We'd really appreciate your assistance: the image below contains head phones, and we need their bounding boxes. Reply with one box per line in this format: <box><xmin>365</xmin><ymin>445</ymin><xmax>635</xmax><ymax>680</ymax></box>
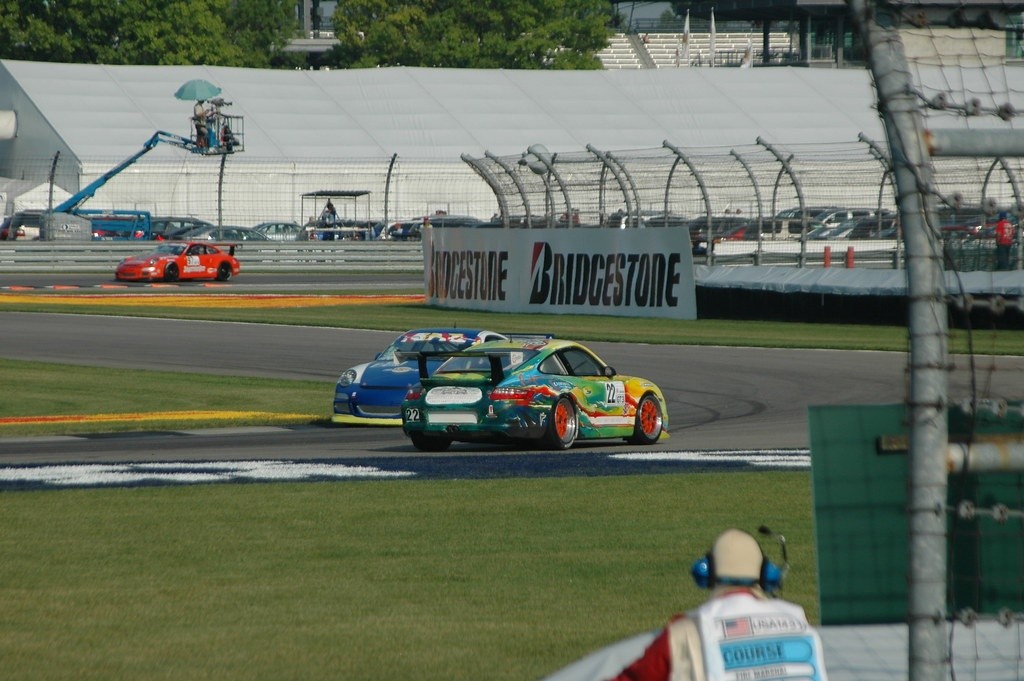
<box><xmin>690</xmin><ymin>529</ymin><xmax>781</xmax><ymax>592</ymax></box>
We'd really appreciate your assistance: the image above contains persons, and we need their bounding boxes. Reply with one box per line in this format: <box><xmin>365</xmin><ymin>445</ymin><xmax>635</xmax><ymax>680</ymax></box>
<box><xmin>421</xmin><ymin>217</ymin><xmax>433</xmax><ymax>243</ymax></box>
<box><xmin>326</xmin><ymin>198</ymin><xmax>336</xmax><ymax>228</ymax></box>
<box><xmin>611</xmin><ymin>527</ymin><xmax>827</xmax><ymax>681</ymax></box>
<box><xmin>996</xmin><ymin>212</ymin><xmax>1014</xmax><ymax>270</ymax></box>
<box><xmin>194</xmin><ymin>99</ymin><xmax>206</xmax><ymax>148</ymax></box>
<box><xmin>644</xmin><ymin>33</ymin><xmax>651</xmax><ymax>43</ymax></box>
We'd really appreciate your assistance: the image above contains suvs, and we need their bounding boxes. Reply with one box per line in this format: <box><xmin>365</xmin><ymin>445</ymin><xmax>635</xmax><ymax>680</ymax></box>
<box><xmin>0</xmin><ymin>209</ymin><xmax>45</xmax><ymax>241</ymax></box>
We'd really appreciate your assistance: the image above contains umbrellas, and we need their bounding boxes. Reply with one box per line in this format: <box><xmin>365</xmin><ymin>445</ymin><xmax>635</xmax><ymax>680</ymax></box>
<box><xmin>174</xmin><ymin>80</ymin><xmax>221</xmax><ymax>101</ymax></box>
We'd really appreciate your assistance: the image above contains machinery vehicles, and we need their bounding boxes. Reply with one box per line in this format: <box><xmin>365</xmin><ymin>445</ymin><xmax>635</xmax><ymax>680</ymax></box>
<box><xmin>53</xmin><ymin>97</ymin><xmax>244</xmax><ymax>243</ymax></box>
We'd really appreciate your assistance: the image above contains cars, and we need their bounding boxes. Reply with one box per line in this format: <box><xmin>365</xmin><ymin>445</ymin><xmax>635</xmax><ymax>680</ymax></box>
<box><xmin>380</xmin><ymin>213</ymin><xmax>518</xmax><ymax>243</ymax></box>
<box><xmin>150</xmin><ymin>213</ymin><xmax>302</xmax><ymax>243</ymax></box>
<box><xmin>607</xmin><ymin>204</ymin><xmax>1019</xmax><ymax>253</ymax></box>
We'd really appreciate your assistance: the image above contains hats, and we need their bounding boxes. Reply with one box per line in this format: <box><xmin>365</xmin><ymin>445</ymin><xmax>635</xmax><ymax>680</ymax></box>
<box><xmin>1000</xmin><ymin>212</ymin><xmax>1007</xmax><ymax>219</ymax></box>
<box><xmin>709</xmin><ymin>527</ymin><xmax>763</xmax><ymax>585</ymax></box>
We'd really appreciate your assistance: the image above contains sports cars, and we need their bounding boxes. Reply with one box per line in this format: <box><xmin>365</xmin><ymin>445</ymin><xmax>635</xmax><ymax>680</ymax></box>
<box><xmin>115</xmin><ymin>242</ymin><xmax>243</xmax><ymax>283</ymax></box>
<box><xmin>331</xmin><ymin>325</ymin><xmax>526</xmax><ymax>427</ymax></box>
<box><xmin>392</xmin><ymin>336</ymin><xmax>669</xmax><ymax>453</ymax></box>
